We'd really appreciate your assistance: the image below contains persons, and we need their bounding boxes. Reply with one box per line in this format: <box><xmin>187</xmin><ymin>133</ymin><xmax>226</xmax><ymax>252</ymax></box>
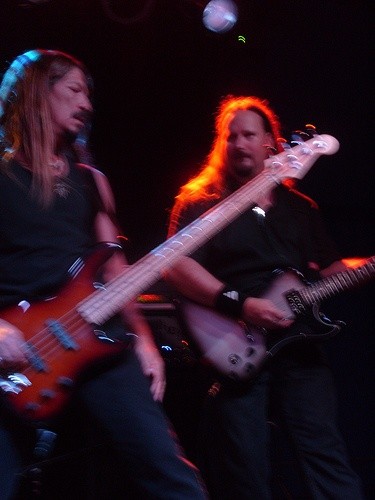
<box><xmin>162</xmin><ymin>94</ymin><xmax>375</xmax><ymax>500</ymax></box>
<box><xmin>1</xmin><ymin>52</ymin><xmax>206</xmax><ymax>500</ymax></box>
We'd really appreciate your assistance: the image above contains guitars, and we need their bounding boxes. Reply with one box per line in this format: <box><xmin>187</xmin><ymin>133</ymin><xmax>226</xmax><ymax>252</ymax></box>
<box><xmin>176</xmin><ymin>252</ymin><xmax>375</xmax><ymax>386</ymax></box>
<box><xmin>1</xmin><ymin>125</ymin><xmax>340</xmax><ymax>416</ymax></box>
